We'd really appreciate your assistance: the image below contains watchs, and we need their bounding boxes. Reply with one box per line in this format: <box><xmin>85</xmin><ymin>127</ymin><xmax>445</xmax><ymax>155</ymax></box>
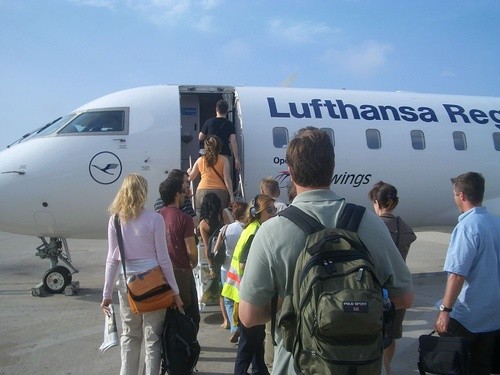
<box><xmin>439</xmin><ymin>304</ymin><xmax>453</xmax><ymax>312</ymax></box>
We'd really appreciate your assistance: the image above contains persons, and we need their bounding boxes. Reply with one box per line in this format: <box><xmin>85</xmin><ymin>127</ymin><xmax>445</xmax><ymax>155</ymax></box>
<box><xmin>100</xmin><ymin>174</ymin><xmax>184</xmax><ymax>375</ymax></box>
<box><xmin>154</xmin><ymin>98</ymin><xmax>297</xmax><ymax>374</ymax></box>
<box><xmin>436</xmin><ymin>172</ymin><xmax>500</xmax><ymax>375</ymax></box>
<box><xmin>237</xmin><ymin>124</ymin><xmax>414</xmax><ymax>375</ymax></box>
<box><xmin>369</xmin><ymin>180</ymin><xmax>417</xmax><ymax>374</ymax></box>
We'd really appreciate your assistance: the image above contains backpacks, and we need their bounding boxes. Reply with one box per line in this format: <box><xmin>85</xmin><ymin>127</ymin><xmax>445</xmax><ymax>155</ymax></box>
<box><xmin>161</xmin><ymin>304</ymin><xmax>200</xmax><ymax>375</ymax></box>
<box><xmin>274</xmin><ymin>203</ymin><xmax>396</xmax><ymax>375</ymax></box>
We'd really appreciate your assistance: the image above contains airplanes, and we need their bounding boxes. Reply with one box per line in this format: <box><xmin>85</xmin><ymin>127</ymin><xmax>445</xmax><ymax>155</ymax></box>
<box><xmin>0</xmin><ymin>84</ymin><xmax>500</xmax><ymax>298</ymax></box>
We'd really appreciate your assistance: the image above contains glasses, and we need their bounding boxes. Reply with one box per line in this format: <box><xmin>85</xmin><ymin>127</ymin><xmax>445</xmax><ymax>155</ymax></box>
<box><xmin>266</xmin><ymin>207</ymin><xmax>277</xmax><ymax>214</ymax></box>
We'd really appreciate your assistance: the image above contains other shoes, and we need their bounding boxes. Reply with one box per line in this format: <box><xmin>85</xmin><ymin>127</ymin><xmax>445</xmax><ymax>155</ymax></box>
<box><xmin>230</xmin><ymin>329</ymin><xmax>241</xmax><ymax>343</ymax></box>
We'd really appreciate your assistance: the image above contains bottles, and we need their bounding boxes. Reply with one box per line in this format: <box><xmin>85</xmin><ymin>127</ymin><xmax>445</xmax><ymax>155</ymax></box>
<box><xmin>211</xmin><ymin>237</ymin><xmax>217</xmax><ymax>253</ymax></box>
<box><xmin>382</xmin><ymin>288</ymin><xmax>393</xmax><ymax>349</ymax></box>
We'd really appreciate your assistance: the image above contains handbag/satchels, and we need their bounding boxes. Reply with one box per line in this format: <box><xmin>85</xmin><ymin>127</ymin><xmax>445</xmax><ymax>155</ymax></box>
<box><xmin>125</xmin><ymin>265</ymin><xmax>176</xmax><ymax>316</ymax></box>
<box><xmin>213</xmin><ymin>223</ymin><xmax>229</xmax><ymax>266</ymax></box>
<box><xmin>222</xmin><ymin>207</ymin><xmax>235</xmax><ymax>224</ymax></box>
<box><xmin>418</xmin><ymin>328</ymin><xmax>470</xmax><ymax>375</ymax></box>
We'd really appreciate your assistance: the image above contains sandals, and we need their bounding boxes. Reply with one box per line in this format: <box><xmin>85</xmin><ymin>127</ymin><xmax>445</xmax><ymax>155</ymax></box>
<box><xmin>223</xmin><ymin>320</ymin><xmax>230</xmax><ymax>328</ymax></box>
